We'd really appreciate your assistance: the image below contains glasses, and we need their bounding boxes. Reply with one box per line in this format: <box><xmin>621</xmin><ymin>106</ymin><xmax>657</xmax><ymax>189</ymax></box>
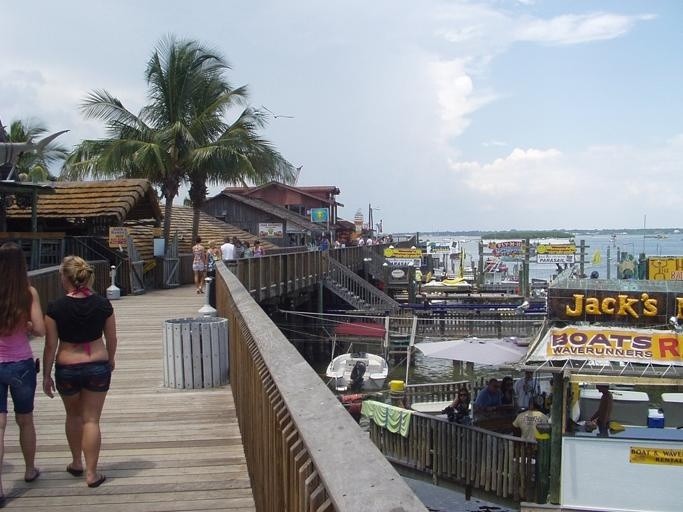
<box><xmin>459</xmin><ymin>393</ymin><xmax>466</xmax><ymax>396</ymax></box>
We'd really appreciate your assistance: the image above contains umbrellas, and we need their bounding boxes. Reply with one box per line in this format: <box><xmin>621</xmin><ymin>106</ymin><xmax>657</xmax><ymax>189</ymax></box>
<box><xmin>414</xmin><ymin>338</ymin><xmax>522</xmax><ymax>426</ymax></box>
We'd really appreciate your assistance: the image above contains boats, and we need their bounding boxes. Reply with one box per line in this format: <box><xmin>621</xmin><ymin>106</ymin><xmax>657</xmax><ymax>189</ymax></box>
<box><xmin>393</xmin><ymin>257</ymin><xmax>549</xmax><ymax>303</ymax></box>
<box><xmin>326</xmin><ymin>352</ymin><xmax>388</xmax><ymax>392</ymax></box>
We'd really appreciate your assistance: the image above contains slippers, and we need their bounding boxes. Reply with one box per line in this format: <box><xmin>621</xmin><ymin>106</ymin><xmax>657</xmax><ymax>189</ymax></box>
<box><xmin>66</xmin><ymin>463</ymin><xmax>83</xmax><ymax>475</ymax></box>
<box><xmin>88</xmin><ymin>474</ymin><xmax>106</xmax><ymax>488</ymax></box>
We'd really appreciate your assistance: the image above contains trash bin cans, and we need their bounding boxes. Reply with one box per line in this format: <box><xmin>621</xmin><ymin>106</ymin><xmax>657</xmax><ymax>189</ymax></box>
<box><xmin>164</xmin><ymin>317</ymin><xmax>230</xmax><ymax>389</ymax></box>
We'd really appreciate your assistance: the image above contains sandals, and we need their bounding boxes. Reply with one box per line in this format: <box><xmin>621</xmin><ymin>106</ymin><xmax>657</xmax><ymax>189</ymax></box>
<box><xmin>25</xmin><ymin>467</ymin><xmax>39</xmax><ymax>483</ymax></box>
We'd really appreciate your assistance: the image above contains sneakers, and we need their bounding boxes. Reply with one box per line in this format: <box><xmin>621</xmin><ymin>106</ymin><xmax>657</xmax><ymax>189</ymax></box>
<box><xmin>196</xmin><ymin>286</ymin><xmax>203</xmax><ymax>293</ymax></box>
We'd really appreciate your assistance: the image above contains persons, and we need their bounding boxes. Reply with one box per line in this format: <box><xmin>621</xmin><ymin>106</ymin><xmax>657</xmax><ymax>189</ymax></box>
<box><xmin>192</xmin><ymin>236</ymin><xmax>264</xmax><ymax>294</ymax></box>
<box><xmin>441</xmin><ymin>371</ymin><xmax>613</xmax><ymax>440</ymax></box>
<box><xmin>318</xmin><ymin>234</ymin><xmax>393</xmax><ymax>250</ymax></box>
<box><xmin>0</xmin><ymin>242</ymin><xmax>117</xmax><ymax>502</ymax></box>
